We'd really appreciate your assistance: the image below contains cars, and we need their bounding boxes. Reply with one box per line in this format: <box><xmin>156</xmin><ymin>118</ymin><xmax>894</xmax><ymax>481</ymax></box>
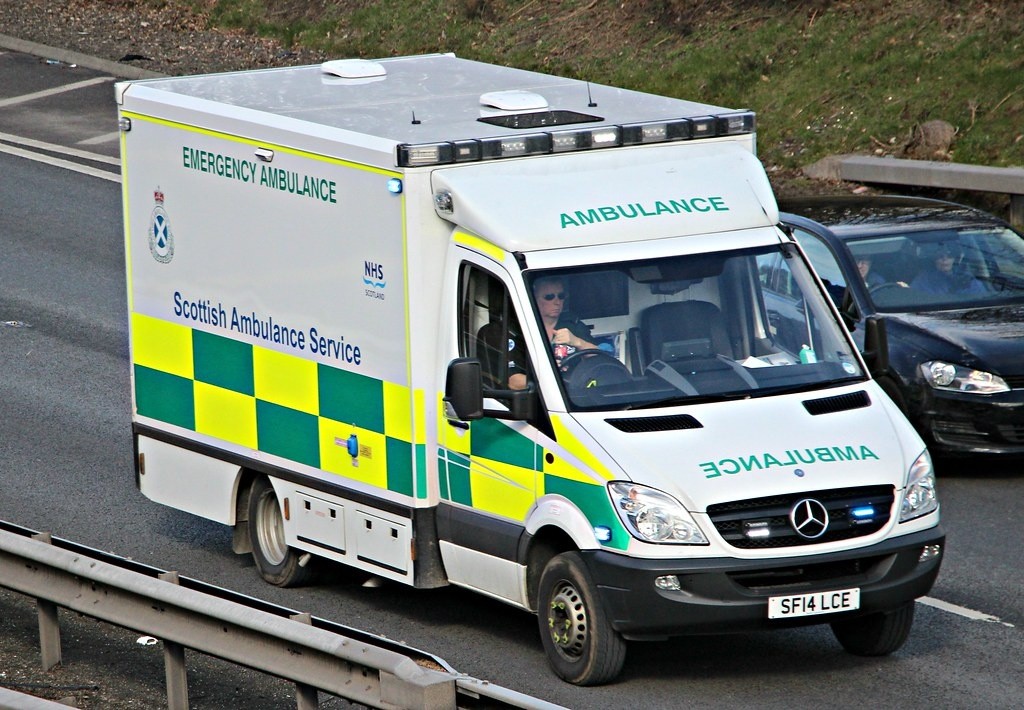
<box><xmin>756</xmin><ymin>195</ymin><xmax>1024</xmax><ymax>479</ymax></box>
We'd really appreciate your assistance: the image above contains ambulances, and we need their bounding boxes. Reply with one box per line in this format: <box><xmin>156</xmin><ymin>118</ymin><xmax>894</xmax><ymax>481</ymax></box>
<box><xmin>115</xmin><ymin>53</ymin><xmax>946</xmax><ymax>686</ymax></box>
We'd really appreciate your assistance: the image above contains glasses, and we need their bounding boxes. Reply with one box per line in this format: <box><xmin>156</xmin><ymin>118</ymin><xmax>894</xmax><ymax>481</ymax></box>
<box><xmin>543</xmin><ymin>292</ymin><xmax>567</xmax><ymax>301</ymax></box>
<box><xmin>934</xmin><ymin>252</ymin><xmax>955</xmax><ymax>260</ymax></box>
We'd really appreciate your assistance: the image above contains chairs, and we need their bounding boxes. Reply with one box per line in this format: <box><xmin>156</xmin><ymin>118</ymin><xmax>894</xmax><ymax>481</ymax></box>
<box><xmin>477</xmin><ymin>319</ymin><xmax>592</xmax><ymax>389</ymax></box>
<box><xmin>630</xmin><ymin>300</ymin><xmax>733</xmax><ymax>378</ymax></box>
<box><xmin>892</xmin><ymin>246</ymin><xmax>929</xmax><ymax>284</ymax></box>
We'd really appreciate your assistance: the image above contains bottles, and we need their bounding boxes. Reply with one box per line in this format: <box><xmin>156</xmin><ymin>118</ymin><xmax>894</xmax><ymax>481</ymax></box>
<box><xmin>555</xmin><ymin>344</ymin><xmax>567</xmax><ymax>363</ymax></box>
<box><xmin>799</xmin><ymin>344</ymin><xmax>817</xmax><ymax>364</ymax></box>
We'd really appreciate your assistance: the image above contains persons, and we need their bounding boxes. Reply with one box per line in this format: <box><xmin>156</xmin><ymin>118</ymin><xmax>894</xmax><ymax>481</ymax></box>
<box><xmin>826</xmin><ymin>244</ymin><xmax>987</xmax><ymax>305</ymax></box>
<box><xmin>507</xmin><ymin>277</ymin><xmax>616</xmax><ymax>391</ymax></box>
